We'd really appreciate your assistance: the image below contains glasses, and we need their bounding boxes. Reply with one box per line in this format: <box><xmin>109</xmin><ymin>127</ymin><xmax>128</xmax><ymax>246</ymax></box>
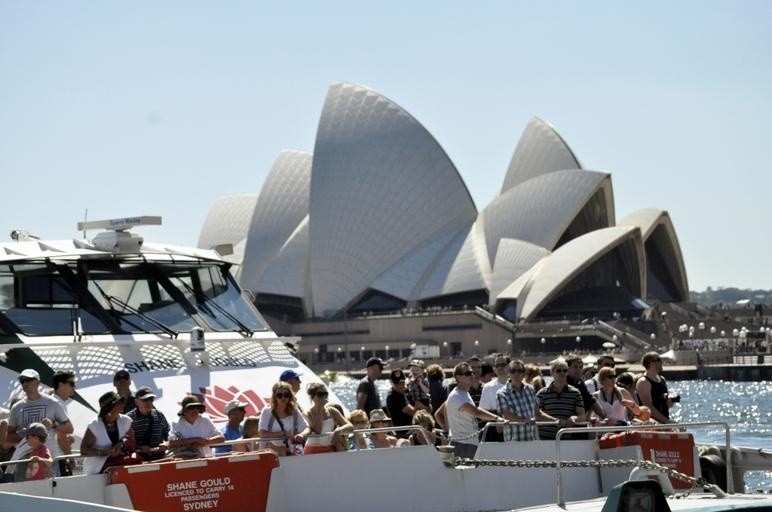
<box><xmin>276</xmin><ymin>392</ymin><xmax>291</xmax><ymax>398</ymax></box>
<box><xmin>462</xmin><ymin>363</ymin><xmax>569</xmax><ymax>378</ymax></box>
<box><xmin>317</xmin><ymin>391</ymin><xmax>329</xmax><ymax>397</ymax></box>
<box><xmin>605</xmin><ymin>362</ymin><xmax>614</xmax><ymax>368</ymax></box>
<box><xmin>607</xmin><ymin>374</ymin><xmax>617</xmax><ymax>379</ymax></box>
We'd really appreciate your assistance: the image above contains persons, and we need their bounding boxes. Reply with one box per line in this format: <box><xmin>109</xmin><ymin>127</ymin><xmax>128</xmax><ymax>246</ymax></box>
<box><xmin>353</xmin><ymin>351</ymin><xmax>687</xmax><ymax>461</ymax></box>
<box><xmin>0</xmin><ymin>370</ymin><xmax>354</xmax><ymax>484</ymax></box>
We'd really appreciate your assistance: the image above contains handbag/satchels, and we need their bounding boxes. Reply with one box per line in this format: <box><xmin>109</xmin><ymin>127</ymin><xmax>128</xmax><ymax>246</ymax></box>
<box><xmin>107</xmin><ymin>436</ymin><xmax>141</xmax><ymax>465</ymax></box>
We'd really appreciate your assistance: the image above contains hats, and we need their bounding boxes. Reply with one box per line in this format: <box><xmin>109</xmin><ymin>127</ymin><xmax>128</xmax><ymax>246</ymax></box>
<box><xmin>98</xmin><ymin>370</ymin><xmax>157</xmax><ymax>417</ymax></box>
<box><xmin>370</xmin><ymin>409</ymin><xmax>391</xmax><ymax>423</ymax></box>
<box><xmin>225</xmin><ymin>400</ymin><xmax>248</xmax><ymax>414</ymax></box>
<box><xmin>177</xmin><ymin>395</ymin><xmax>205</xmax><ymax>416</ymax></box>
<box><xmin>17</xmin><ymin>367</ymin><xmax>41</xmax><ymax>384</ymax></box>
<box><xmin>280</xmin><ymin>370</ymin><xmax>302</xmax><ymax>382</ymax></box>
<box><xmin>19</xmin><ymin>423</ymin><xmax>48</xmax><ymax>438</ymax></box>
<box><xmin>367</xmin><ymin>357</ymin><xmax>424</xmax><ymax>384</ymax></box>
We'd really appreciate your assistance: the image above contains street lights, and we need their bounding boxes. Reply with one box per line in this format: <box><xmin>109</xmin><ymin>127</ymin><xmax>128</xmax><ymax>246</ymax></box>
<box><xmin>333</xmin><ymin>344</ymin><xmax>342</xmax><ymax>363</ymax></box>
<box><xmin>648</xmin><ymin>332</ymin><xmax>656</xmax><ymax>351</ymax></box>
<box><xmin>659</xmin><ymin>310</ymin><xmax>668</xmax><ymax>324</ymax></box>
<box><xmin>384</xmin><ymin>344</ymin><xmax>390</xmax><ymax>360</ymax></box>
<box><xmin>408</xmin><ymin>341</ymin><xmax>416</xmax><ymax>359</ymax></box>
<box><xmin>358</xmin><ymin>344</ymin><xmax>366</xmax><ymax>361</ymax></box>
<box><xmin>673</xmin><ymin>317</ymin><xmax>771</xmax><ymax>352</ymax></box>
<box><xmin>541</xmin><ymin>335</ymin><xmax>546</xmax><ymax>356</ymax></box>
<box><xmin>444</xmin><ymin>341</ymin><xmax>448</xmax><ymax>358</ymax></box>
<box><xmin>473</xmin><ymin>339</ymin><xmax>480</xmax><ymax>357</ymax></box>
<box><xmin>612</xmin><ymin>332</ymin><xmax>618</xmax><ymax>351</ymax></box>
<box><xmin>507</xmin><ymin>337</ymin><xmax>512</xmax><ymax>355</ymax></box>
<box><xmin>313</xmin><ymin>346</ymin><xmax>319</xmax><ymax>362</ymax></box>
<box><xmin>574</xmin><ymin>332</ymin><xmax>581</xmax><ymax>354</ymax></box>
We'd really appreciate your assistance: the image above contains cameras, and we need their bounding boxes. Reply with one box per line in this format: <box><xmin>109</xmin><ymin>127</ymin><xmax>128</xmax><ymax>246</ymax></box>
<box><xmin>663</xmin><ymin>392</ymin><xmax>681</xmax><ymax>402</ymax></box>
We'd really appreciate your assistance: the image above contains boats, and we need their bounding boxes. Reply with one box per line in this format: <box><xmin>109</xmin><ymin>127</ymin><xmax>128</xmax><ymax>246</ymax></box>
<box><xmin>0</xmin><ymin>212</ymin><xmax>772</xmax><ymax>512</ymax></box>
